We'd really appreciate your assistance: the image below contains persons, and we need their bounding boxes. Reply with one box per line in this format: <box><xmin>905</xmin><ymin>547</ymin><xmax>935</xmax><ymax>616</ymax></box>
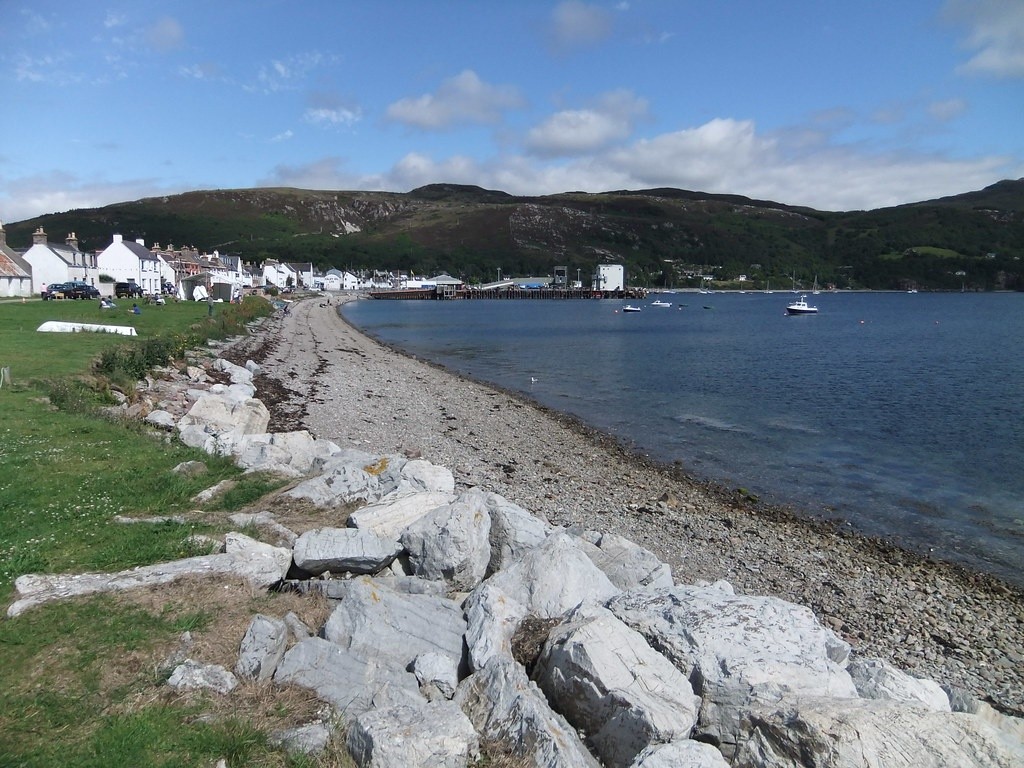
<box><xmin>207</xmin><ymin>294</ymin><xmax>213</xmax><ymax>317</ymax></box>
<box><xmin>100</xmin><ymin>295</ymin><xmax>116</xmax><ymax>309</ymax></box>
<box><xmin>232</xmin><ymin>288</ymin><xmax>244</xmax><ymax>306</ymax></box>
<box><xmin>128</xmin><ymin>302</ymin><xmax>141</xmax><ymax>314</ymax></box>
<box><xmin>460</xmin><ymin>284</ymin><xmax>648</xmax><ymax>299</ymax></box>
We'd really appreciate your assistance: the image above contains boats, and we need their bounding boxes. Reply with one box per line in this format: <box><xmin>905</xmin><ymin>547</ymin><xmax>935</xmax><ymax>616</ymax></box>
<box><xmin>785</xmin><ymin>295</ymin><xmax>818</xmax><ymax>316</ymax></box>
<box><xmin>651</xmin><ymin>300</ymin><xmax>673</xmax><ymax>308</ymax></box>
<box><xmin>37</xmin><ymin>321</ymin><xmax>139</xmax><ymax>337</ymax></box>
<box><xmin>621</xmin><ymin>305</ymin><xmax>642</xmax><ymax>312</ymax></box>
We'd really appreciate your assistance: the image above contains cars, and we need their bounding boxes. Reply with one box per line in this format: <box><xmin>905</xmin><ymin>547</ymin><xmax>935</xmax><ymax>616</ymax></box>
<box><xmin>46</xmin><ymin>283</ymin><xmax>72</xmax><ymax>298</ymax></box>
<box><xmin>69</xmin><ymin>285</ymin><xmax>100</xmax><ymax>298</ymax></box>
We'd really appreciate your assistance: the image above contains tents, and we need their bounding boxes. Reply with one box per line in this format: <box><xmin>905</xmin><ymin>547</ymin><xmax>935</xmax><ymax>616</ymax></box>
<box><xmin>175</xmin><ymin>271</ymin><xmax>240</xmax><ymax>303</ymax></box>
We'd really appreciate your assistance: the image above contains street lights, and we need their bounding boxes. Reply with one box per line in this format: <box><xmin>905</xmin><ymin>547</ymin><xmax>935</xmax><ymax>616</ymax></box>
<box><xmin>497</xmin><ymin>268</ymin><xmax>501</xmax><ymax>281</ymax></box>
<box><xmin>576</xmin><ymin>268</ymin><xmax>581</xmax><ymax>291</ymax></box>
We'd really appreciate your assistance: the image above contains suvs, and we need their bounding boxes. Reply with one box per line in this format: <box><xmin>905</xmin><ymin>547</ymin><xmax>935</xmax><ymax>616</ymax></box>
<box><xmin>116</xmin><ymin>282</ymin><xmax>143</xmax><ymax>299</ymax></box>
<box><xmin>64</xmin><ymin>281</ymin><xmax>86</xmax><ymax>291</ymax></box>
